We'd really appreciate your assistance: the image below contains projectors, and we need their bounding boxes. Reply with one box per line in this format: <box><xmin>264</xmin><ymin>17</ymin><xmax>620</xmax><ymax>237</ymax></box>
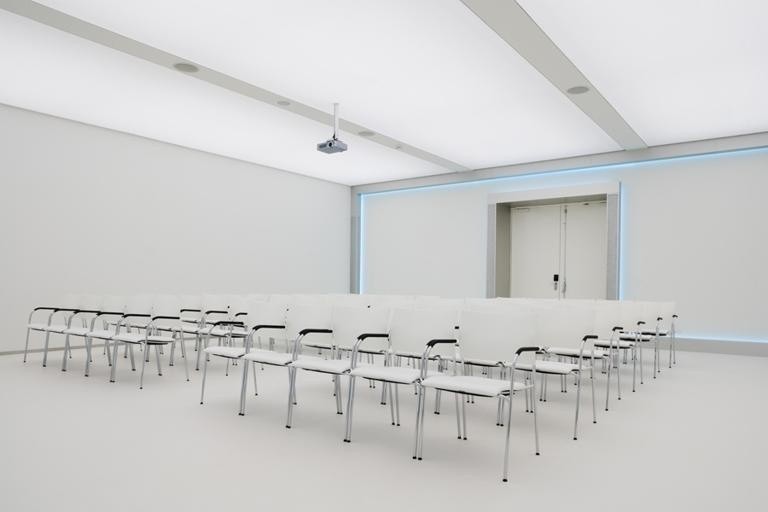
<box><xmin>316</xmin><ymin>140</ymin><xmax>347</xmax><ymax>154</ymax></box>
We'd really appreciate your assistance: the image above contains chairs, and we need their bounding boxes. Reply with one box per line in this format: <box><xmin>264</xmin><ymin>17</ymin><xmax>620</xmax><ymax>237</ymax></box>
<box><xmin>21</xmin><ymin>294</ymin><xmax>679</xmax><ymax>483</ymax></box>
